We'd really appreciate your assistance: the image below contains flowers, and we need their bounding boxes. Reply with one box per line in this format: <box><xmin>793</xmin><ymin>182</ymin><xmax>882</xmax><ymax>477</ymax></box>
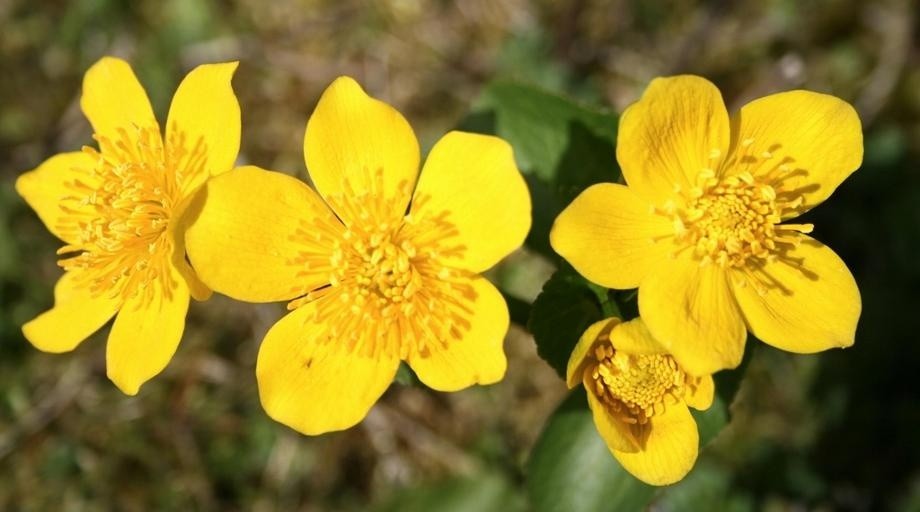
<box><xmin>10</xmin><ymin>53</ymin><xmax>906</xmax><ymax>511</ymax></box>
